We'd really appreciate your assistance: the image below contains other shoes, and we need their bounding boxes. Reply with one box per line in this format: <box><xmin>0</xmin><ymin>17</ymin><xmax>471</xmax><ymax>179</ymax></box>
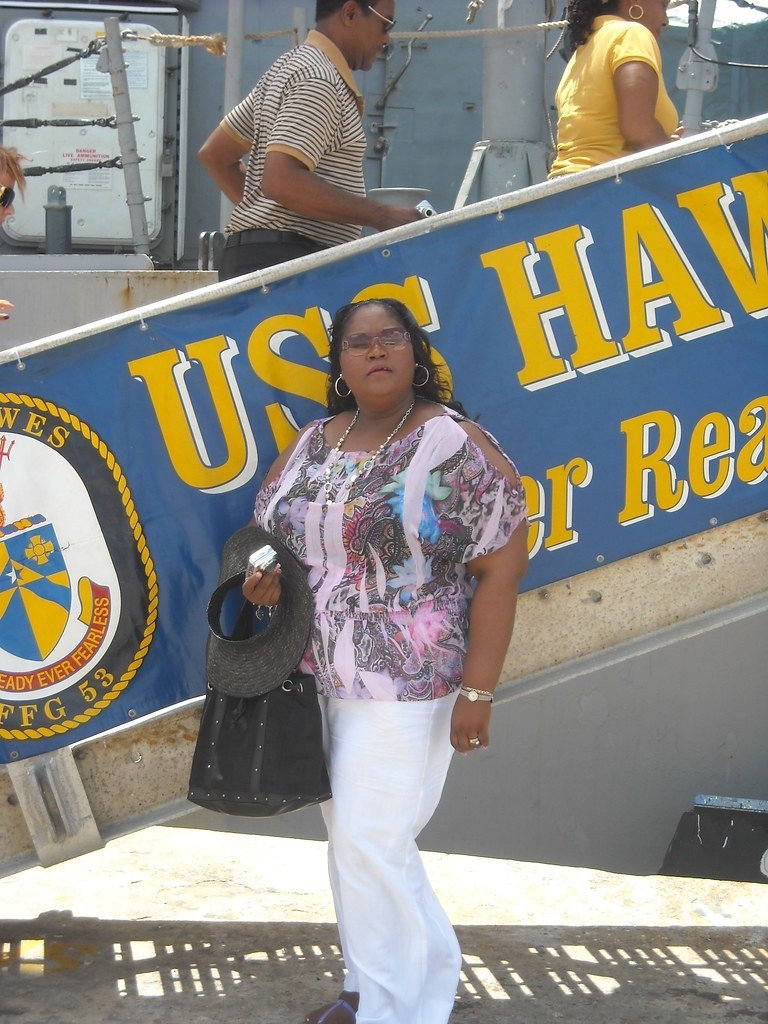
<box><xmin>302</xmin><ymin>990</ymin><xmax>360</xmax><ymax>1024</ymax></box>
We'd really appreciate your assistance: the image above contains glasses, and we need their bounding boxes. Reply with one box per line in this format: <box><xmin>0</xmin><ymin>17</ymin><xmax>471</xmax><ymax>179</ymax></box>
<box><xmin>0</xmin><ymin>183</ymin><xmax>15</xmax><ymax>209</ymax></box>
<box><xmin>357</xmin><ymin>0</ymin><xmax>397</xmax><ymax>32</ymax></box>
<box><xmin>341</xmin><ymin>327</ymin><xmax>411</xmax><ymax>356</ymax></box>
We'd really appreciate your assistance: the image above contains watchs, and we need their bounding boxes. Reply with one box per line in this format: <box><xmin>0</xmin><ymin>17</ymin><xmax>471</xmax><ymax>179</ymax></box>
<box><xmin>459</xmin><ymin>691</ymin><xmax>495</xmax><ymax>702</ymax></box>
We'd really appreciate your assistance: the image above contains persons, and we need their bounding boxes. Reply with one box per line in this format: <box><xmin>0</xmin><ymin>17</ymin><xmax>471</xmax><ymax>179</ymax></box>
<box><xmin>242</xmin><ymin>298</ymin><xmax>532</xmax><ymax>1024</ymax></box>
<box><xmin>0</xmin><ymin>146</ymin><xmax>26</xmax><ymax>321</ymax></box>
<box><xmin>199</xmin><ymin>0</ymin><xmax>422</xmax><ymax>282</ymax></box>
<box><xmin>547</xmin><ymin>0</ymin><xmax>684</xmax><ymax>180</ymax></box>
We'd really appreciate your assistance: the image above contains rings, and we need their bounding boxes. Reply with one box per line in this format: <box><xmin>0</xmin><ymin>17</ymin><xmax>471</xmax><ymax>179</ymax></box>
<box><xmin>469</xmin><ymin>736</ymin><xmax>480</xmax><ymax>745</ymax></box>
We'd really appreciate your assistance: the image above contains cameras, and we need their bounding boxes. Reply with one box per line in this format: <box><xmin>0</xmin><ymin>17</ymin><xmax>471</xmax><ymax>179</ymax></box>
<box><xmin>245</xmin><ymin>545</ymin><xmax>277</xmax><ymax>581</ymax></box>
<box><xmin>416</xmin><ymin>200</ymin><xmax>437</xmax><ymax>218</ymax></box>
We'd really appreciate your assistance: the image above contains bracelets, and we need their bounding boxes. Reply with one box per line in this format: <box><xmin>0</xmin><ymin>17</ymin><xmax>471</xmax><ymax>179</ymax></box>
<box><xmin>461</xmin><ymin>686</ymin><xmax>491</xmax><ymax>696</ymax></box>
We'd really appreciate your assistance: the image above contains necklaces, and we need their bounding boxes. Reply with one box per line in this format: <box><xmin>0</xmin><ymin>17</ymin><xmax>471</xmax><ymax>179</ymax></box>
<box><xmin>324</xmin><ymin>400</ymin><xmax>416</xmax><ymax>510</ymax></box>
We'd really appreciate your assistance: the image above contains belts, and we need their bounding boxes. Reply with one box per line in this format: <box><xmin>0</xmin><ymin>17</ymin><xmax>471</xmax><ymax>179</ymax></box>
<box><xmin>221</xmin><ymin>229</ymin><xmax>328</xmax><ymax>252</ymax></box>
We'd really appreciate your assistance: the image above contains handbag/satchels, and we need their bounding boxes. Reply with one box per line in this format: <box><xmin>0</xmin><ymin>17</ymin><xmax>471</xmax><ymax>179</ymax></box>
<box><xmin>187</xmin><ymin>598</ymin><xmax>332</xmax><ymax>817</ymax></box>
